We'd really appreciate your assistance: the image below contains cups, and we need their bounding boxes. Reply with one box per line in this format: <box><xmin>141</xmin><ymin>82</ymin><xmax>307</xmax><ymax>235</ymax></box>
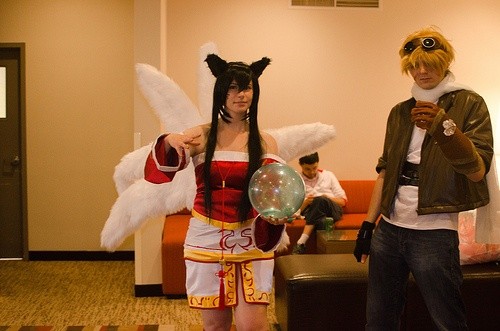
<box><xmin>416</xmin><ymin>100</ymin><xmax>435</xmax><ymax>129</ymax></box>
<box><xmin>324</xmin><ymin>218</ymin><xmax>333</xmax><ymax>233</ymax></box>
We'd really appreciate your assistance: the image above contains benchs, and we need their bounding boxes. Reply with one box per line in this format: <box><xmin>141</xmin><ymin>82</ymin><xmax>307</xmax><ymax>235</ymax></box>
<box><xmin>274</xmin><ymin>255</ymin><xmax>500</xmax><ymax>331</ymax></box>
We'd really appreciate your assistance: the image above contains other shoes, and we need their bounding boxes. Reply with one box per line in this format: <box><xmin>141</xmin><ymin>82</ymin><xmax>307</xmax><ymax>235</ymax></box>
<box><xmin>291</xmin><ymin>243</ymin><xmax>305</xmax><ymax>254</ymax></box>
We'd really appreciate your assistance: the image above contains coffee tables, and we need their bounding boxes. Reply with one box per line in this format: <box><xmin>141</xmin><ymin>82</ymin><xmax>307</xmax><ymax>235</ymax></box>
<box><xmin>316</xmin><ymin>230</ymin><xmax>362</xmax><ymax>255</ymax></box>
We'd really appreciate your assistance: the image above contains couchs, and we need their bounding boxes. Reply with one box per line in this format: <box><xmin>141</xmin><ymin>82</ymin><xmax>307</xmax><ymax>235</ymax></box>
<box><xmin>161</xmin><ymin>180</ymin><xmax>375</xmax><ymax>299</ymax></box>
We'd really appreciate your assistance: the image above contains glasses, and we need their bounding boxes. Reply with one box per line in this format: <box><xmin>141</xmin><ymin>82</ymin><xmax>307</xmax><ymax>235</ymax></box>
<box><xmin>403</xmin><ymin>37</ymin><xmax>447</xmax><ymax>53</ymax></box>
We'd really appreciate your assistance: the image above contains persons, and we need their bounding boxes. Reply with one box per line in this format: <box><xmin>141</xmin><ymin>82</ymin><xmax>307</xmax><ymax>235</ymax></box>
<box><xmin>353</xmin><ymin>26</ymin><xmax>494</xmax><ymax>331</ymax></box>
<box><xmin>144</xmin><ymin>54</ymin><xmax>297</xmax><ymax>331</ymax></box>
<box><xmin>287</xmin><ymin>152</ymin><xmax>348</xmax><ymax>255</ymax></box>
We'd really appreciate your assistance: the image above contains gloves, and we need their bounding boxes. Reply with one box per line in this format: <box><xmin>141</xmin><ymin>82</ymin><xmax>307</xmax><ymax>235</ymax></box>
<box><xmin>410</xmin><ymin>100</ymin><xmax>441</xmax><ymax>130</ymax></box>
